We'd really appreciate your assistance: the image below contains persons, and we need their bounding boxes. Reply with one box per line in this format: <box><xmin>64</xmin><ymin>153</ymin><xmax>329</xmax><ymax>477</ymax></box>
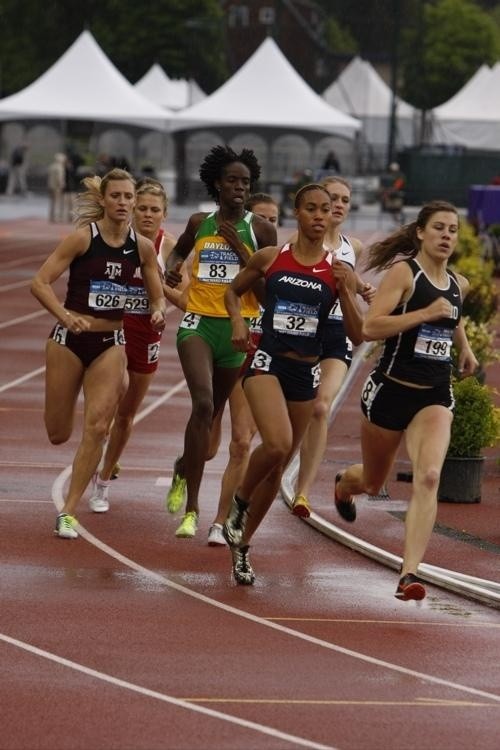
<box><xmin>334</xmin><ymin>202</ymin><xmax>482</xmax><ymax>605</ymax></box>
<box><xmin>3</xmin><ymin>143</ymin><xmax>410</xmax><ymax>586</ymax></box>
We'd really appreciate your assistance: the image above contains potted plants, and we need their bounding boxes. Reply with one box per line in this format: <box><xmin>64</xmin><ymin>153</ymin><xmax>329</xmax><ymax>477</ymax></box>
<box><xmin>437</xmin><ymin>218</ymin><xmax>500</xmax><ymax>504</ymax></box>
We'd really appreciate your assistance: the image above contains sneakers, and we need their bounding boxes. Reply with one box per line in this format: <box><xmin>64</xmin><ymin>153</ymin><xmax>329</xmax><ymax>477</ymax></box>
<box><xmin>88</xmin><ymin>461</ymin><xmax>120</xmax><ymax>513</ymax></box>
<box><xmin>166</xmin><ymin>456</ymin><xmax>199</xmax><ymax>538</ymax></box>
<box><xmin>54</xmin><ymin>513</ymin><xmax>78</xmax><ymax>539</ymax></box>
<box><xmin>207</xmin><ymin>486</ymin><xmax>256</xmax><ymax>586</ymax></box>
<box><xmin>335</xmin><ymin>470</ymin><xmax>356</xmax><ymax>522</ymax></box>
<box><xmin>394</xmin><ymin>572</ymin><xmax>425</xmax><ymax>600</ymax></box>
<box><xmin>288</xmin><ymin>495</ymin><xmax>310</xmax><ymax>519</ymax></box>
